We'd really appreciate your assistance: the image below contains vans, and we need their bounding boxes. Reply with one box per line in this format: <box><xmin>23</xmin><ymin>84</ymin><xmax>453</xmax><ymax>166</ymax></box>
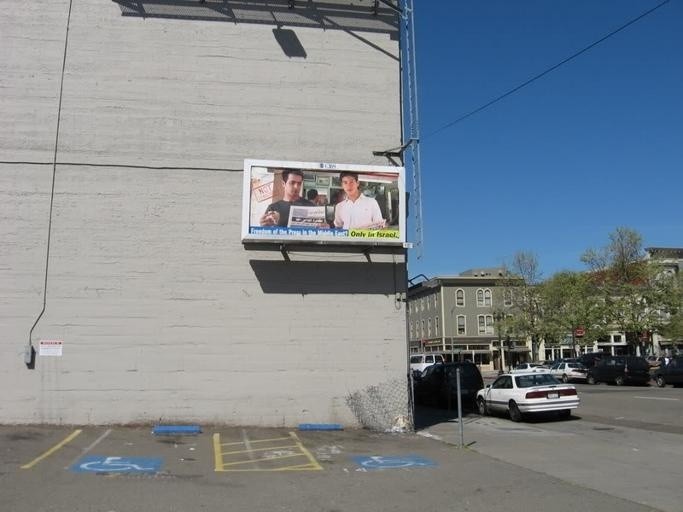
<box><xmin>411</xmin><ymin>354</ymin><xmax>447</xmax><ymax>379</ymax></box>
<box><xmin>413</xmin><ymin>361</ymin><xmax>484</xmax><ymax>408</ymax></box>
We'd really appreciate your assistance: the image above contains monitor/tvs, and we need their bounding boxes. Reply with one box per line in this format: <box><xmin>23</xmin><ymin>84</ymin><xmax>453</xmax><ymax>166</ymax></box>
<box><xmin>241</xmin><ymin>158</ymin><xmax>406</xmax><ymax>246</ymax></box>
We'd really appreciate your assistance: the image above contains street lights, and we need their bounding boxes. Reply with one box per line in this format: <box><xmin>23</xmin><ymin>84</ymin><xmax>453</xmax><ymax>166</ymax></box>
<box><xmin>492</xmin><ymin>310</ymin><xmax>506</xmax><ymax>376</ymax></box>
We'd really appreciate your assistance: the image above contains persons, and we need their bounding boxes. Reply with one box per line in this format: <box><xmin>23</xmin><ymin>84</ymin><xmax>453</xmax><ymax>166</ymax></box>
<box><xmin>331</xmin><ymin>172</ymin><xmax>385</xmax><ymax>230</ymax></box>
<box><xmin>302</xmin><ymin>185</ymin><xmax>320</xmax><ymax>205</ymax></box>
<box><xmin>258</xmin><ymin>169</ymin><xmax>329</xmax><ymax>228</ymax></box>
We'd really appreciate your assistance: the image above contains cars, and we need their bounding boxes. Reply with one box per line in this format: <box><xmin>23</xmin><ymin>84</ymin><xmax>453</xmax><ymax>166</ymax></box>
<box><xmin>475</xmin><ymin>372</ymin><xmax>579</xmax><ymax>421</ymax></box>
<box><xmin>509</xmin><ymin>351</ymin><xmax>682</xmax><ymax>387</ymax></box>
